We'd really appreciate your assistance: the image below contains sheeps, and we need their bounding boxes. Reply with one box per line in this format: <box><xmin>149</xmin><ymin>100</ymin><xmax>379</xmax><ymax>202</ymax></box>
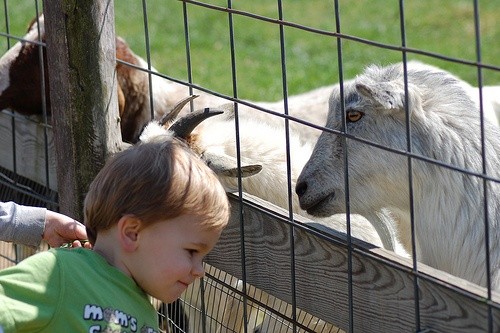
<box><xmin>0</xmin><ymin>9</ymin><xmax>500</xmax><ymax>333</ymax></box>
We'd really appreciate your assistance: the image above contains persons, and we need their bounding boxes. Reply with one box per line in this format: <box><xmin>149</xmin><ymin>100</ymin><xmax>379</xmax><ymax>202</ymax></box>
<box><xmin>0</xmin><ymin>201</ymin><xmax>93</xmax><ymax>250</ymax></box>
<box><xmin>0</xmin><ymin>132</ymin><xmax>232</xmax><ymax>333</ymax></box>
